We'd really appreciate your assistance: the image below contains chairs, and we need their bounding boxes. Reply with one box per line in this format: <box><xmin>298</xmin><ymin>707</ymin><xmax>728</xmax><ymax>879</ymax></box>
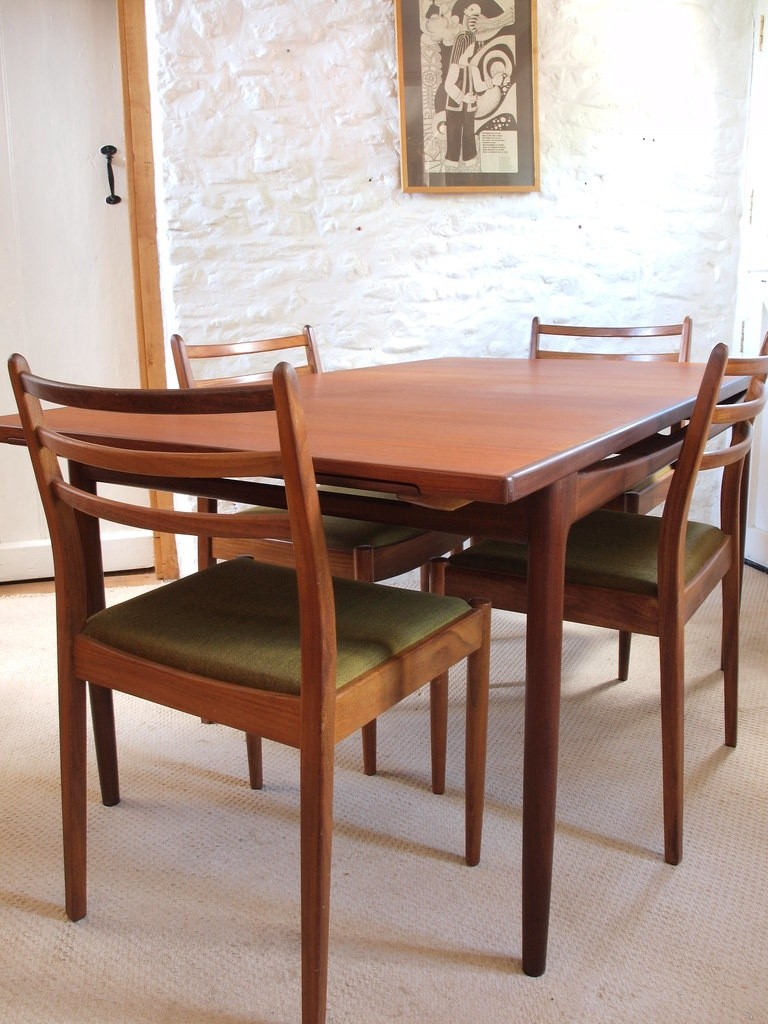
<box><xmin>429</xmin><ymin>332</ymin><xmax>768</xmax><ymax>863</ymax></box>
<box><xmin>6</xmin><ymin>351</ymin><xmax>493</xmax><ymax>1024</ymax></box>
<box><xmin>531</xmin><ymin>316</ymin><xmax>694</xmax><ymax>682</ymax></box>
<box><xmin>168</xmin><ymin>323</ymin><xmax>471</xmax><ymax>595</ymax></box>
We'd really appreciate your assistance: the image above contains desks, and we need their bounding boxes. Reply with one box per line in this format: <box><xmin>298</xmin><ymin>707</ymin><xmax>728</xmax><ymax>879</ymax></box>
<box><xmin>0</xmin><ymin>356</ymin><xmax>751</xmax><ymax>978</ymax></box>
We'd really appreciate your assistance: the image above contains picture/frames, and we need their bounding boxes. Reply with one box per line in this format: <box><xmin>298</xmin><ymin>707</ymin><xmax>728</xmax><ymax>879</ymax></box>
<box><xmin>395</xmin><ymin>0</ymin><xmax>539</xmax><ymax>194</ymax></box>
